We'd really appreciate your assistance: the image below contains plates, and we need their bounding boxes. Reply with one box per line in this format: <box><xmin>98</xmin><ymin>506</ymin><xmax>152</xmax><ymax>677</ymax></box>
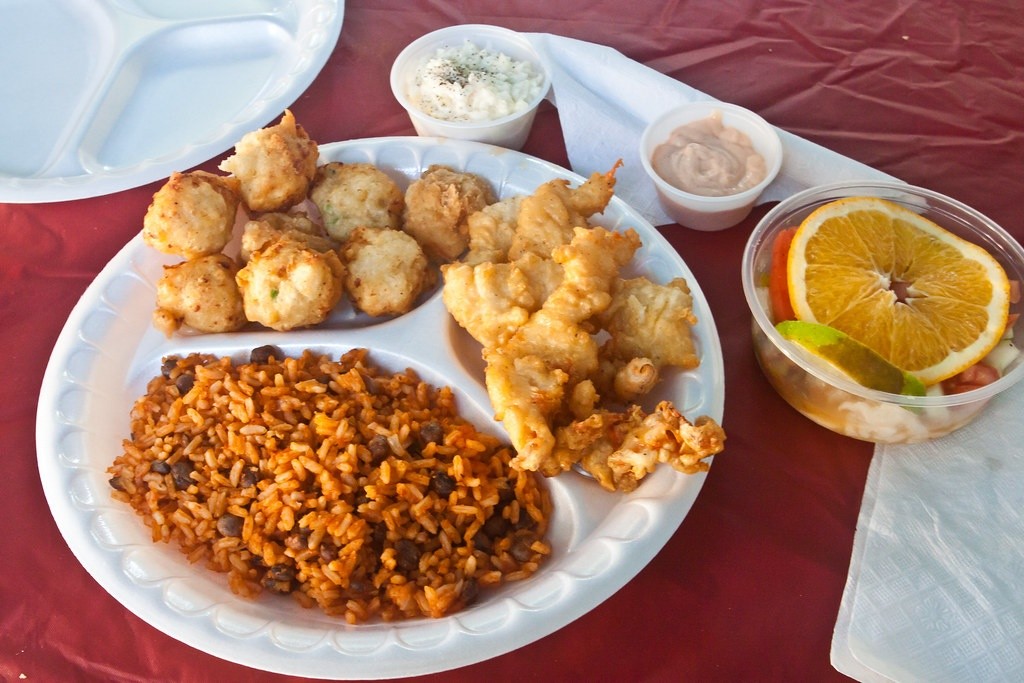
<box><xmin>1</xmin><ymin>0</ymin><xmax>347</xmax><ymax>206</ymax></box>
<box><xmin>30</xmin><ymin>132</ymin><xmax>728</xmax><ymax>680</ymax></box>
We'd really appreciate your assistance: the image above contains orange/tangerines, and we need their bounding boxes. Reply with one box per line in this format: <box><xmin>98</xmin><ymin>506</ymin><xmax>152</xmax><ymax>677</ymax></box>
<box><xmin>787</xmin><ymin>196</ymin><xmax>1011</xmax><ymax>385</ymax></box>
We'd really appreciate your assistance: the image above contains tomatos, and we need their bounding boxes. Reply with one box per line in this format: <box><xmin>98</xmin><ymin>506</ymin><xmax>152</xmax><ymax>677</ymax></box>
<box><xmin>771</xmin><ymin>226</ymin><xmax>801</xmax><ymax>322</ymax></box>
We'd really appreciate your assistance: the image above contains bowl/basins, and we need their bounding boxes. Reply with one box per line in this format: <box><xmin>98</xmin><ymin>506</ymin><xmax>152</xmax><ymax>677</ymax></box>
<box><xmin>740</xmin><ymin>179</ymin><xmax>1024</xmax><ymax>443</ymax></box>
<box><xmin>390</xmin><ymin>24</ymin><xmax>552</xmax><ymax>152</ymax></box>
<box><xmin>638</xmin><ymin>101</ymin><xmax>783</xmax><ymax>232</ymax></box>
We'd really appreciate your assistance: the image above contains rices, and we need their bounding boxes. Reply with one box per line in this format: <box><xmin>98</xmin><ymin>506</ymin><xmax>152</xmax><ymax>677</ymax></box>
<box><xmin>107</xmin><ymin>346</ymin><xmax>554</xmax><ymax>623</ymax></box>
